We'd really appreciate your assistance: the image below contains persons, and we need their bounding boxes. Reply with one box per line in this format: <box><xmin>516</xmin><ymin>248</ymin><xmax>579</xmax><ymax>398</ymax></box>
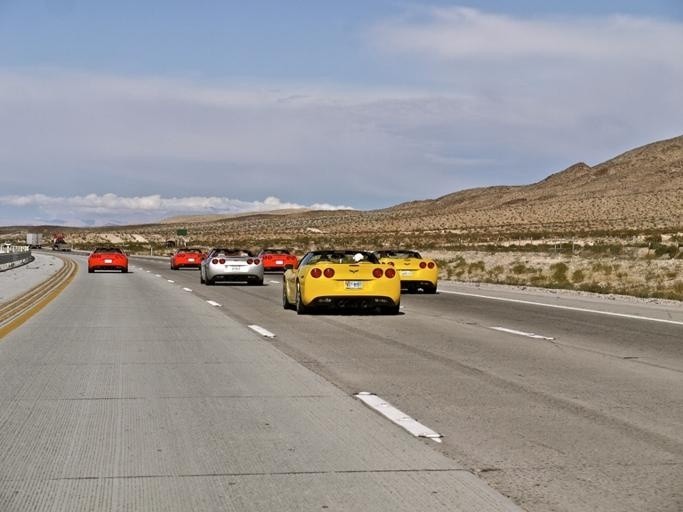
<box><xmin>320</xmin><ymin>255</ymin><xmax>328</xmax><ymax>259</ymax></box>
<box><xmin>353</xmin><ymin>253</ymin><xmax>364</xmax><ymax>262</ymax></box>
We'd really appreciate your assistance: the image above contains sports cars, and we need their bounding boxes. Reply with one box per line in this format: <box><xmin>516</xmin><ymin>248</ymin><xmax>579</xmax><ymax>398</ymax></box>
<box><xmin>170</xmin><ymin>247</ymin><xmax>439</xmax><ymax>315</ymax></box>
<box><xmin>87</xmin><ymin>246</ymin><xmax>128</xmax><ymax>273</ymax></box>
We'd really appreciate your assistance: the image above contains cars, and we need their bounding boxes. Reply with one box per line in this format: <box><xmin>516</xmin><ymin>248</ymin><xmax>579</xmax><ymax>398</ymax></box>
<box><xmin>3</xmin><ymin>241</ymin><xmax>12</xmax><ymax>248</ymax></box>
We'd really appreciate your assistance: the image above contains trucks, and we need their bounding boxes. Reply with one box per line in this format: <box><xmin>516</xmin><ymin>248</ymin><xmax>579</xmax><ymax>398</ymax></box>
<box><xmin>26</xmin><ymin>233</ymin><xmax>43</xmax><ymax>249</ymax></box>
<box><xmin>51</xmin><ymin>234</ymin><xmax>71</xmax><ymax>252</ymax></box>
